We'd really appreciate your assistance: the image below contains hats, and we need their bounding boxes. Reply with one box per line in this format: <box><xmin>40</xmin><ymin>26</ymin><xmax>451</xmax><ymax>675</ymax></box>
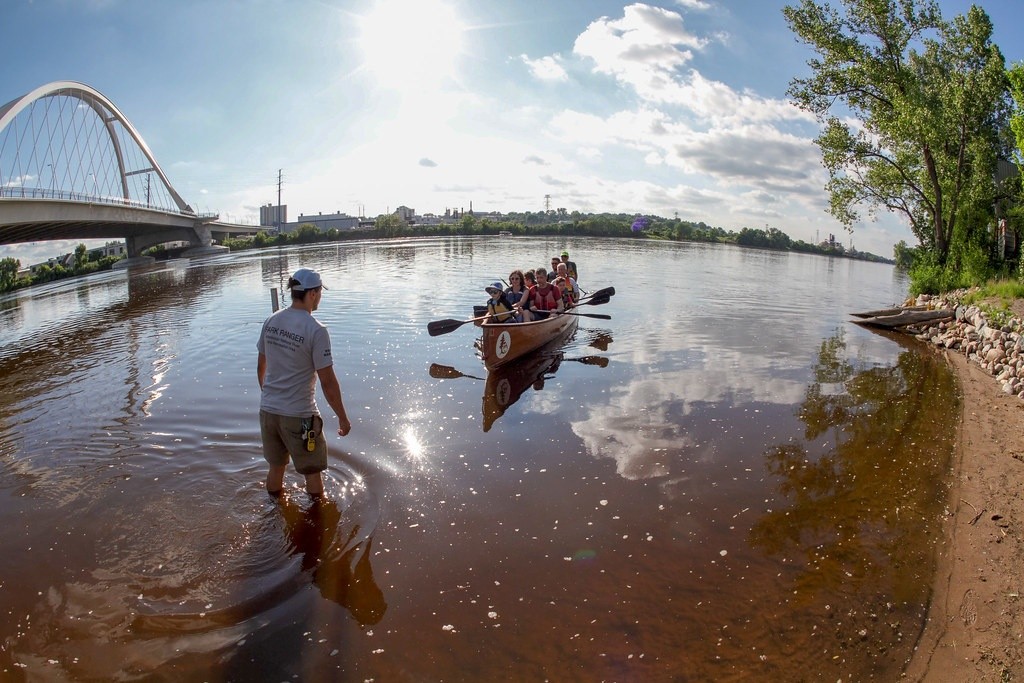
<box><xmin>287</xmin><ymin>268</ymin><xmax>330</xmax><ymax>291</ymax></box>
<box><xmin>561</xmin><ymin>251</ymin><xmax>569</xmax><ymax>257</ymax></box>
<box><xmin>485</xmin><ymin>282</ymin><xmax>506</xmax><ymax>297</ymax></box>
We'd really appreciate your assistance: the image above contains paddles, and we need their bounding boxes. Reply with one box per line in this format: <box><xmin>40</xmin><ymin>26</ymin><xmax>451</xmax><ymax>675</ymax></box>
<box><xmin>576</xmin><ymin>286</ymin><xmax>616</xmax><ymax>299</ymax></box>
<box><xmin>563</xmin><ymin>293</ymin><xmax>610</xmax><ymax>309</ymax></box>
<box><xmin>534</xmin><ymin>309</ymin><xmax>612</xmax><ymax>320</ymax></box>
<box><xmin>426</xmin><ymin>310</ymin><xmax>519</xmax><ymax>337</ymax></box>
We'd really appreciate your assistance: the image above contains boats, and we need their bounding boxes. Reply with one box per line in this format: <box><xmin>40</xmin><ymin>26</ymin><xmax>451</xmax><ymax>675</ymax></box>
<box><xmin>480</xmin><ymin>295</ymin><xmax>579</xmax><ymax>370</ymax></box>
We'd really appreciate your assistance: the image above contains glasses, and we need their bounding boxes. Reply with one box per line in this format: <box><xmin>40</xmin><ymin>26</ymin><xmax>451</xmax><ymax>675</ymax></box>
<box><xmin>510</xmin><ymin>277</ymin><xmax>519</xmax><ymax>281</ymax></box>
<box><xmin>552</xmin><ymin>263</ymin><xmax>557</xmax><ymax>265</ymax></box>
<box><xmin>488</xmin><ymin>290</ymin><xmax>500</xmax><ymax>295</ymax></box>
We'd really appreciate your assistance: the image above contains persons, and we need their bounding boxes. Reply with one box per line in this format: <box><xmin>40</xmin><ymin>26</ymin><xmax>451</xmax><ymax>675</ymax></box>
<box><xmin>485</xmin><ymin>251</ymin><xmax>580</xmax><ymax>324</ymax></box>
<box><xmin>256</xmin><ymin>267</ymin><xmax>351</xmax><ymax>495</ymax></box>
